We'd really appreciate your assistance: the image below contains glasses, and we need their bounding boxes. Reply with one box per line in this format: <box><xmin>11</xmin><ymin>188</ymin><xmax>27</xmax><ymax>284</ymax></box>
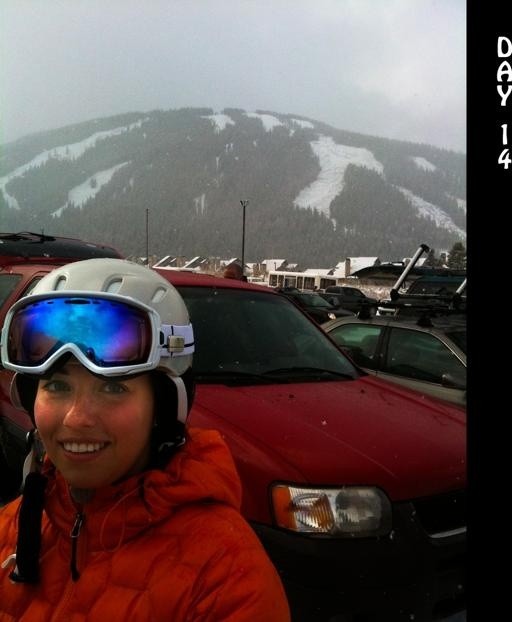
<box><xmin>1</xmin><ymin>290</ymin><xmax>195</xmax><ymax>381</ymax></box>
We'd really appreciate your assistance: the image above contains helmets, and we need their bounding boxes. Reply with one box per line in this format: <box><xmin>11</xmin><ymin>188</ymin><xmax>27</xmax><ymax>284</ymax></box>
<box><xmin>7</xmin><ymin>257</ymin><xmax>196</xmax><ymax>420</ymax></box>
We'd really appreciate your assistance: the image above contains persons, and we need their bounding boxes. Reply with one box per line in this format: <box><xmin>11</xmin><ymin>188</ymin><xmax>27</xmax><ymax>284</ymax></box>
<box><xmin>0</xmin><ymin>258</ymin><xmax>291</xmax><ymax>622</ymax></box>
